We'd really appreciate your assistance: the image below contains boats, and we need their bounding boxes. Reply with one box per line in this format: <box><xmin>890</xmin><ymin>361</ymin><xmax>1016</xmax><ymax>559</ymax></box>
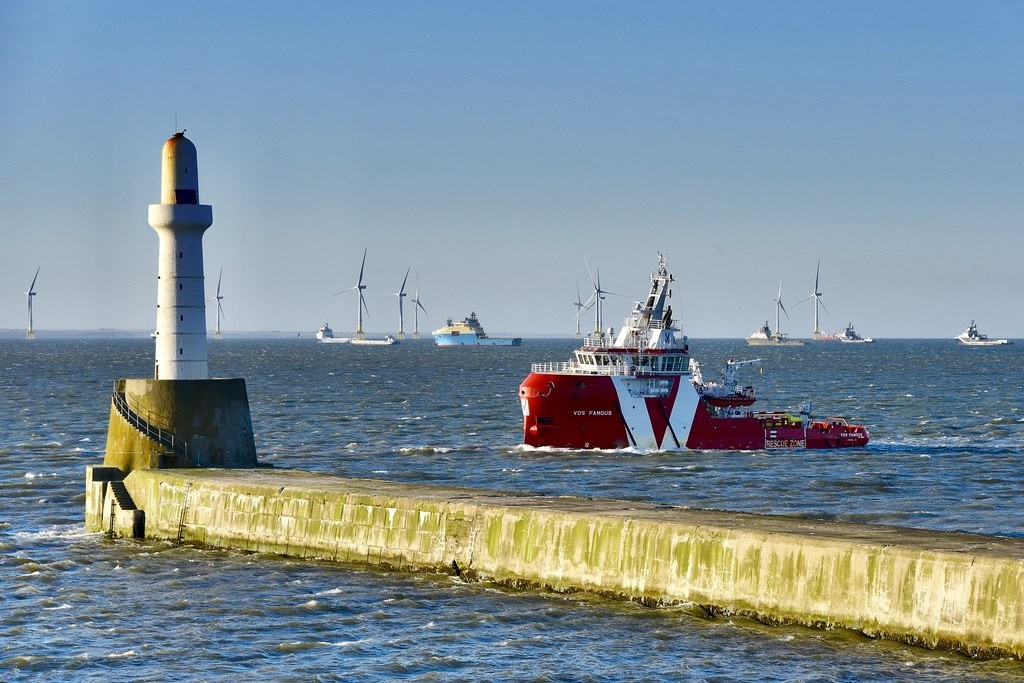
<box><xmin>432</xmin><ymin>312</ymin><xmax>522</xmax><ymax>346</ymax></box>
<box><xmin>955</xmin><ymin>319</ymin><xmax>1013</xmax><ymax>346</ymax></box>
<box><xmin>838</xmin><ymin>321</ymin><xmax>873</xmax><ymax>343</ymax></box>
<box><xmin>518</xmin><ymin>250</ymin><xmax>869</xmax><ymax>452</ymax></box>
<box><xmin>316</xmin><ymin>322</ymin><xmax>400</xmax><ymax>345</ymax></box>
<box><xmin>744</xmin><ymin>321</ymin><xmax>806</xmax><ymax>346</ymax></box>
